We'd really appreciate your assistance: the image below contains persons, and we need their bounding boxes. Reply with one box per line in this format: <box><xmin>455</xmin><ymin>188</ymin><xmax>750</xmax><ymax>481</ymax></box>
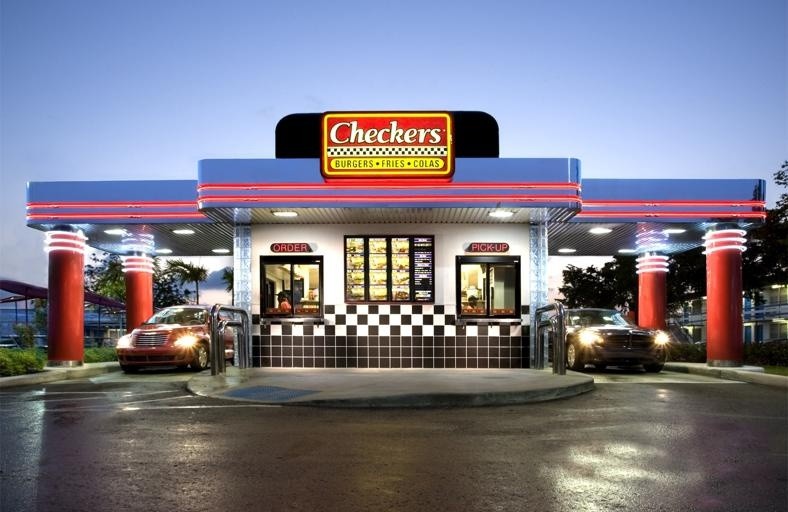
<box><xmin>466</xmin><ymin>295</ymin><xmax>480</xmax><ymax>309</ymax></box>
<box><xmin>274</xmin><ymin>291</ymin><xmax>291</xmax><ymax>312</ymax></box>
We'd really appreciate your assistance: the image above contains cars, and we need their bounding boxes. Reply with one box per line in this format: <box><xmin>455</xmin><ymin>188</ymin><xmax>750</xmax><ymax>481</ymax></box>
<box><xmin>115</xmin><ymin>304</ymin><xmax>234</xmax><ymax>371</ymax></box>
<box><xmin>548</xmin><ymin>307</ymin><xmax>668</xmax><ymax>373</ymax></box>
